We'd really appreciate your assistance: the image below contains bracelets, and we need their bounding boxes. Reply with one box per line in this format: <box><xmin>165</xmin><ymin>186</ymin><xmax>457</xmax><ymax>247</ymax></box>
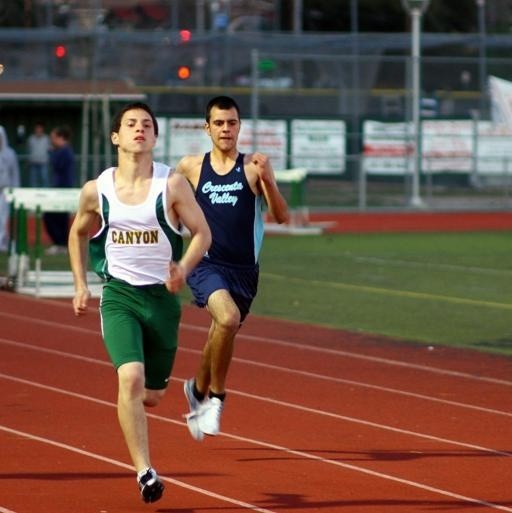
<box><xmin>178</xmin><ymin>258</ymin><xmax>191</xmax><ymax>278</ymax></box>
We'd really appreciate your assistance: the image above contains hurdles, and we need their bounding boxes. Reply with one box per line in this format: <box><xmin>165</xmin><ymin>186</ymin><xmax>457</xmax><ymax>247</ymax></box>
<box><xmin>2</xmin><ymin>186</ymin><xmax>103</xmax><ymax>298</ymax></box>
<box><xmin>263</xmin><ymin>169</ymin><xmax>338</xmax><ymax>234</ymax></box>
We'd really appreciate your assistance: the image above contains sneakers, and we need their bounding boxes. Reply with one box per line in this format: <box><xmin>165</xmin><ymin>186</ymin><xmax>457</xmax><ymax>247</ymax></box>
<box><xmin>43</xmin><ymin>244</ymin><xmax>69</xmax><ymax>255</ymax></box>
<box><xmin>136</xmin><ymin>466</ymin><xmax>165</xmax><ymax>504</ymax></box>
<box><xmin>197</xmin><ymin>394</ymin><xmax>224</xmax><ymax>439</ymax></box>
<box><xmin>181</xmin><ymin>375</ymin><xmax>206</xmax><ymax>443</ymax></box>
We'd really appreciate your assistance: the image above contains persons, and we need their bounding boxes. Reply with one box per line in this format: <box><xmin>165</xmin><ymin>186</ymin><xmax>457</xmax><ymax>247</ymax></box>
<box><xmin>172</xmin><ymin>94</ymin><xmax>291</xmax><ymax>443</ymax></box>
<box><xmin>66</xmin><ymin>105</ymin><xmax>215</xmax><ymax>503</ymax></box>
<box><xmin>23</xmin><ymin>122</ymin><xmax>54</xmax><ymax>186</ymax></box>
<box><xmin>42</xmin><ymin>121</ymin><xmax>77</xmax><ymax>256</ymax></box>
<box><xmin>0</xmin><ymin>123</ymin><xmax>24</xmax><ymax>250</ymax></box>
